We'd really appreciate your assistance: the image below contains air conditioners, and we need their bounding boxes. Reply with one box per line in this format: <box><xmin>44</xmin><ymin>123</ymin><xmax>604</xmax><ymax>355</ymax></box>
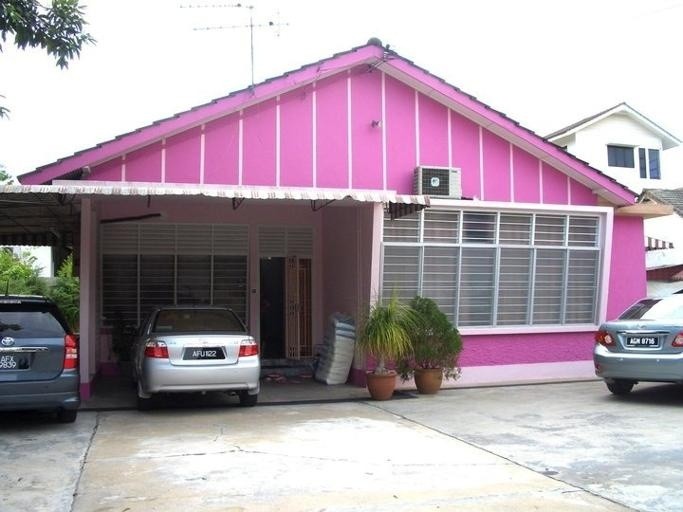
<box><xmin>411</xmin><ymin>165</ymin><xmax>462</xmax><ymax>202</ymax></box>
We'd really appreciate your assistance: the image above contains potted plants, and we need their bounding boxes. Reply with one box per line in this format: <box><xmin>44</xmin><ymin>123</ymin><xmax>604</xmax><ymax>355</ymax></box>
<box><xmin>358</xmin><ymin>286</ymin><xmax>463</xmax><ymax>402</ymax></box>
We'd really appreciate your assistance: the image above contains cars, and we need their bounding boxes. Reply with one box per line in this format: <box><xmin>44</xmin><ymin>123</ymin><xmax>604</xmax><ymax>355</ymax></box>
<box><xmin>129</xmin><ymin>302</ymin><xmax>262</xmax><ymax>410</ymax></box>
<box><xmin>592</xmin><ymin>294</ymin><xmax>683</xmax><ymax>396</ymax></box>
<box><xmin>0</xmin><ymin>293</ymin><xmax>80</xmax><ymax>424</ymax></box>
